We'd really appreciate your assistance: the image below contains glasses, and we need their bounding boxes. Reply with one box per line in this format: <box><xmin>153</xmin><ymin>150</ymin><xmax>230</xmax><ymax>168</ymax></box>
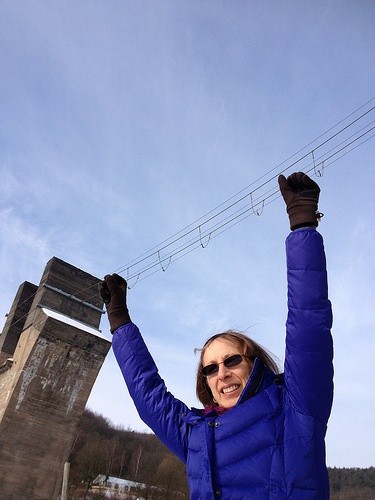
<box><xmin>201</xmin><ymin>354</ymin><xmax>244</xmax><ymax>377</ymax></box>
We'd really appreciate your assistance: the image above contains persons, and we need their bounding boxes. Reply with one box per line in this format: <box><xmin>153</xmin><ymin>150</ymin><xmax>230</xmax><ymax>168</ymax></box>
<box><xmin>100</xmin><ymin>171</ymin><xmax>333</xmax><ymax>500</ymax></box>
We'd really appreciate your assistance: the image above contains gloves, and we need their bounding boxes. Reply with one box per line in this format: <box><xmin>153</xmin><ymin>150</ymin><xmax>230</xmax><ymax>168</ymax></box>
<box><xmin>99</xmin><ymin>273</ymin><xmax>132</xmax><ymax>333</ymax></box>
<box><xmin>278</xmin><ymin>172</ymin><xmax>324</xmax><ymax>231</ymax></box>
